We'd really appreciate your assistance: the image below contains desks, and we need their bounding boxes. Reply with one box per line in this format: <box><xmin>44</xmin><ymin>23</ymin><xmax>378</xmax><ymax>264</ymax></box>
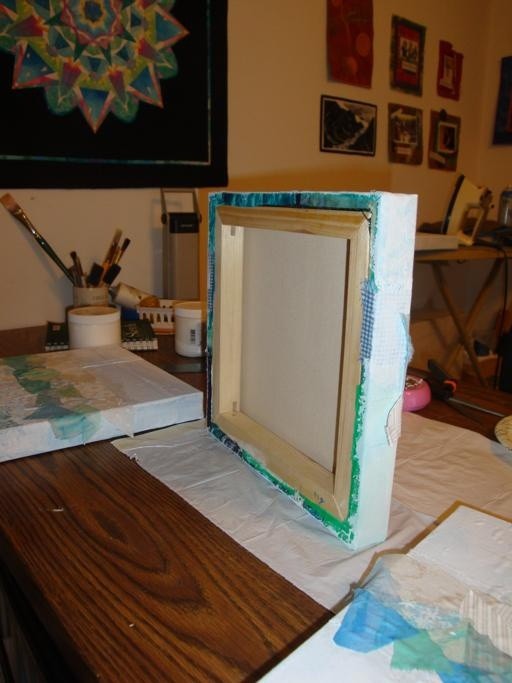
<box><xmin>0</xmin><ymin>321</ymin><xmax>509</xmax><ymax>680</ymax></box>
<box><xmin>407</xmin><ymin>243</ymin><xmax>511</xmax><ymax>390</ymax></box>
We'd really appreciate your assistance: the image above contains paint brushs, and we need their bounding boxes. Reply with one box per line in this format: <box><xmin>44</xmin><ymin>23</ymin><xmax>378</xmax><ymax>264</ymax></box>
<box><xmin>1</xmin><ymin>193</ymin><xmax>130</xmax><ymax>287</ymax></box>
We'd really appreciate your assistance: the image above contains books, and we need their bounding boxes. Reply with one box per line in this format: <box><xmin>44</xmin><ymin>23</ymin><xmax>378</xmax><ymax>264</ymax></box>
<box><xmin>45</xmin><ymin>320</ymin><xmax>160</xmax><ymax>353</ymax></box>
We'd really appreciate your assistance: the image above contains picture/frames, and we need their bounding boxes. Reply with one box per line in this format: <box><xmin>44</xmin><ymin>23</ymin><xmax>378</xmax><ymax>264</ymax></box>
<box><xmin>316</xmin><ymin>91</ymin><xmax>378</xmax><ymax>158</ymax></box>
<box><xmin>388</xmin><ymin>11</ymin><xmax>427</xmax><ymax>99</ymax></box>
<box><xmin>0</xmin><ymin>0</ymin><xmax>234</xmax><ymax>193</ymax></box>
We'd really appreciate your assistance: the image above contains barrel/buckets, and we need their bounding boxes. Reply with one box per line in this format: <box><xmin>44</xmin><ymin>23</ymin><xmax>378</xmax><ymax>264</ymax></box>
<box><xmin>71</xmin><ymin>285</ymin><xmax>113</xmax><ymax>308</ymax></box>
<box><xmin>174</xmin><ymin>300</ymin><xmax>209</xmax><ymax>360</ymax></box>
<box><xmin>68</xmin><ymin>305</ymin><xmax>121</xmax><ymax>350</ymax></box>
